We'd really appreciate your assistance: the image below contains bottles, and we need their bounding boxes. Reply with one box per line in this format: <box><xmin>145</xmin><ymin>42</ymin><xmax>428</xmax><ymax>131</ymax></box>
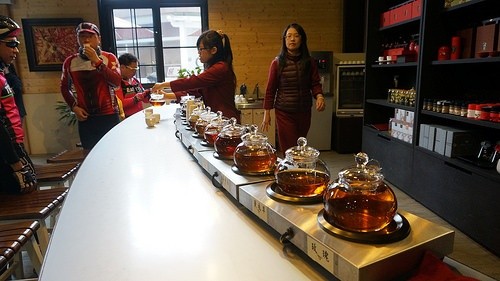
<box><xmin>423</xmin><ymin>95</ymin><xmax>500</xmax><ymax>123</ymax></box>
<box><xmin>240</xmin><ymin>83</ymin><xmax>247</xmax><ymax>96</ymax></box>
<box><xmin>386</xmin><ymin>85</ymin><xmax>417</xmax><ymax>106</ymax></box>
<box><xmin>475</xmin><ymin>138</ymin><xmax>500</xmax><ymax>173</ymax></box>
<box><xmin>378</xmin><ymin>0</ymin><xmax>422</xmax><ymax>65</ymax></box>
<box><xmin>437</xmin><ymin>36</ymin><xmax>460</xmax><ymax>60</ymax></box>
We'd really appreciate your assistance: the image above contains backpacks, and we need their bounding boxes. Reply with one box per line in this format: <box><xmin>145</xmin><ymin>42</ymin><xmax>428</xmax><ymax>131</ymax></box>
<box><xmin>116</xmin><ymin>96</ymin><xmax>125</xmax><ymax>120</ymax></box>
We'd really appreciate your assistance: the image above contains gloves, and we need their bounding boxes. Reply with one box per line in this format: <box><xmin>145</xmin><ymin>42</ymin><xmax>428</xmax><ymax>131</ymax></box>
<box><xmin>12</xmin><ymin>167</ymin><xmax>37</xmax><ymax>197</ymax></box>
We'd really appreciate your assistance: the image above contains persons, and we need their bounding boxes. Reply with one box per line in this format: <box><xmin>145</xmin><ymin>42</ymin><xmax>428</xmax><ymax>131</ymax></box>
<box><xmin>0</xmin><ymin>15</ymin><xmax>39</xmax><ymax>206</ymax></box>
<box><xmin>259</xmin><ymin>22</ymin><xmax>327</xmax><ymax>159</ymax></box>
<box><xmin>112</xmin><ymin>53</ymin><xmax>152</xmax><ymax>116</ymax></box>
<box><xmin>152</xmin><ymin>29</ymin><xmax>240</xmax><ymax>124</ymax></box>
<box><xmin>59</xmin><ymin>22</ymin><xmax>122</xmax><ymax>151</ymax></box>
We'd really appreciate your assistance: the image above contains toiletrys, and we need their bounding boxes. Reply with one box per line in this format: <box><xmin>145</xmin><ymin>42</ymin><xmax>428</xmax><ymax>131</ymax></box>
<box><xmin>239</xmin><ymin>82</ymin><xmax>247</xmax><ymax>100</ymax></box>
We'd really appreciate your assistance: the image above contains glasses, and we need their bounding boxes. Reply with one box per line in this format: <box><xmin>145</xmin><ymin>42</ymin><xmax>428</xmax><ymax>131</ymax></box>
<box><xmin>0</xmin><ymin>39</ymin><xmax>21</xmax><ymax>48</ymax></box>
<box><xmin>75</xmin><ymin>34</ymin><xmax>99</xmax><ymax>39</ymax></box>
<box><xmin>284</xmin><ymin>34</ymin><xmax>301</xmax><ymax>38</ymax></box>
<box><xmin>199</xmin><ymin>47</ymin><xmax>206</xmax><ymax>51</ymax></box>
<box><xmin>125</xmin><ymin>65</ymin><xmax>139</xmax><ymax>71</ymax></box>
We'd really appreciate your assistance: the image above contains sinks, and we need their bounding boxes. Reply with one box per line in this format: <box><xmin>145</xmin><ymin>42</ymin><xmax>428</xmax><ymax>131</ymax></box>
<box><xmin>245</xmin><ymin>98</ymin><xmax>255</xmax><ymax>103</ymax></box>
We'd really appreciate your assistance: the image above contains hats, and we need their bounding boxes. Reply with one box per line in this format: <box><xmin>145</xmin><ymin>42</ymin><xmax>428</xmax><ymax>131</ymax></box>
<box><xmin>76</xmin><ymin>23</ymin><xmax>100</xmax><ymax>34</ymax></box>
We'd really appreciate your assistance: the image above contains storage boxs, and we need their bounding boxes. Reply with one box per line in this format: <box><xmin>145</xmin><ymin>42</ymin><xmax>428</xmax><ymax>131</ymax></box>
<box><xmin>391</xmin><ymin>108</ymin><xmax>474</xmax><ymax>157</ymax></box>
<box><xmin>378</xmin><ymin>0</ymin><xmax>500</xmax><ymax>58</ymax></box>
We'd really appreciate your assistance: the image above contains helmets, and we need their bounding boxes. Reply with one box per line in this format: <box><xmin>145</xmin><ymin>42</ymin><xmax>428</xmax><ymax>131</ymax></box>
<box><xmin>0</xmin><ymin>15</ymin><xmax>21</xmax><ymax>37</ymax></box>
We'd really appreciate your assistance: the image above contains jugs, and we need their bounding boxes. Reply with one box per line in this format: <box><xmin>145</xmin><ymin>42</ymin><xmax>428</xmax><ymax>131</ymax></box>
<box><xmin>180</xmin><ymin>92</ymin><xmax>207</xmax><ymax>130</ymax></box>
<box><xmin>322</xmin><ymin>152</ymin><xmax>398</xmax><ymax>233</ymax></box>
<box><xmin>213</xmin><ymin>117</ymin><xmax>245</xmax><ymax>159</ymax></box>
<box><xmin>203</xmin><ymin>110</ymin><xmax>230</xmax><ymax>146</ymax></box>
<box><xmin>149</xmin><ymin>85</ymin><xmax>166</xmax><ymax>107</ymax></box>
<box><xmin>194</xmin><ymin>106</ymin><xmax>217</xmax><ymax>137</ymax></box>
<box><xmin>233</xmin><ymin>124</ymin><xmax>276</xmax><ymax>173</ymax></box>
<box><xmin>274</xmin><ymin>136</ymin><xmax>330</xmax><ymax>198</ymax></box>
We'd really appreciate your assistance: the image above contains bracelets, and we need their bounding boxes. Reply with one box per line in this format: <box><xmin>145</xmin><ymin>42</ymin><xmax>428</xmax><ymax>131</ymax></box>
<box><xmin>317</xmin><ymin>96</ymin><xmax>325</xmax><ymax>100</ymax></box>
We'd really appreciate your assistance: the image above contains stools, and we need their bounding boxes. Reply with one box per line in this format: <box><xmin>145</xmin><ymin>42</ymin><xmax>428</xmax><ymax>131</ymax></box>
<box><xmin>0</xmin><ymin>143</ymin><xmax>91</xmax><ymax>281</ymax></box>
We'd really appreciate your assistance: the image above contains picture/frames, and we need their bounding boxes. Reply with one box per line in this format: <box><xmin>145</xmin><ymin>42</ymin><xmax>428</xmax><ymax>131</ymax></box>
<box><xmin>22</xmin><ymin>18</ymin><xmax>83</xmax><ymax>72</ymax></box>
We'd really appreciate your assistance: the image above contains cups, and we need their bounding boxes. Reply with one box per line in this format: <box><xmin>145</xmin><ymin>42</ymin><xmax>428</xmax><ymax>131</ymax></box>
<box><xmin>235</xmin><ymin>94</ymin><xmax>246</xmax><ymax>104</ymax></box>
<box><xmin>387</xmin><ymin>109</ymin><xmax>415</xmax><ymax>144</ymax></box>
<box><xmin>82</xmin><ymin>42</ymin><xmax>91</xmax><ymax>50</ymax></box>
<box><xmin>340</xmin><ymin>60</ymin><xmax>366</xmax><ymax>75</ymax></box>
<box><xmin>144</xmin><ymin>108</ymin><xmax>160</xmax><ymax>128</ymax></box>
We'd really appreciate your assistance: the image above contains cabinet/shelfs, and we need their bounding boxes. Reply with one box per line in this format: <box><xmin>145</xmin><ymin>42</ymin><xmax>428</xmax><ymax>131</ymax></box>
<box><xmin>362</xmin><ymin>0</ymin><xmax>424</xmax><ymax>264</ymax></box>
<box><xmin>240</xmin><ymin>108</ymin><xmax>275</xmax><ymax>150</ymax></box>
<box><xmin>412</xmin><ymin>0</ymin><xmax>500</xmax><ymax>257</ymax></box>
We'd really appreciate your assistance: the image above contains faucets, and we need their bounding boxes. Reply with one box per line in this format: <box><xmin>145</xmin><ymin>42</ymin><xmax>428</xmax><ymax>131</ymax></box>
<box><xmin>254</xmin><ymin>82</ymin><xmax>259</xmax><ymax>99</ymax></box>
